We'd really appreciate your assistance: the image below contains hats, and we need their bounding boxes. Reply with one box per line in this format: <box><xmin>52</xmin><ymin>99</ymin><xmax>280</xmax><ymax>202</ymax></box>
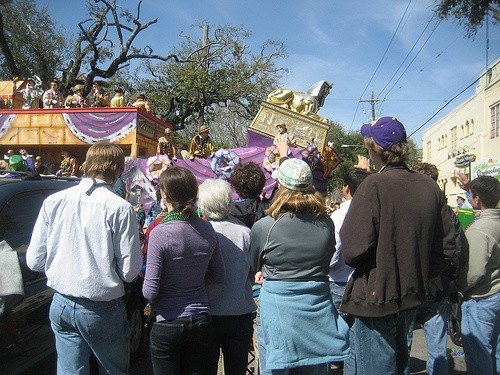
<box><xmin>361</xmin><ymin>117</ymin><xmax>406</xmax><ymax>151</ymax></box>
<box><xmin>276</xmin><ymin>123</ymin><xmax>287</xmax><ymax>129</ymax></box>
<box><xmin>9</xmin><ymin>155</ymin><xmax>27</xmax><ymax>170</ymax></box>
<box><xmin>271</xmin><ymin>158</ymin><xmax>313</xmax><ymax>191</ymax></box>
<box><xmin>200</xmin><ymin>126</ymin><xmax>210</xmax><ymax>133</ymax></box>
<box><xmin>164</xmin><ymin>128</ymin><xmax>173</xmax><ymax>135</ymax></box>
<box><xmin>458</xmin><ymin>196</ymin><xmax>465</xmax><ymax>201</ymax></box>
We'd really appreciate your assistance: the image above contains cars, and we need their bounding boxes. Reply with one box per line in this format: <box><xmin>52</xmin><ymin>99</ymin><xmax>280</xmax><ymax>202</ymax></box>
<box><xmin>0</xmin><ymin>170</ymin><xmax>145</xmax><ymax>375</ymax></box>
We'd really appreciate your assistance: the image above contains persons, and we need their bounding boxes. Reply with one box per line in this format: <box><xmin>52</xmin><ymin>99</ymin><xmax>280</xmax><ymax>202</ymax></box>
<box><xmin>13</xmin><ymin>76</ymin><xmax>44</xmax><ymax>108</ymax></box>
<box><xmin>196</xmin><ymin>177</ymin><xmax>258</xmax><ymax>375</ymax></box>
<box><xmin>324</xmin><ymin>117</ymin><xmax>469</xmax><ymax>375</ymax></box>
<box><xmin>156</xmin><ymin>128</ymin><xmax>178</xmax><ymax>162</ymax></box>
<box><xmin>131</xmin><ymin>94</ymin><xmax>151</xmax><ymax>113</ymax></box>
<box><xmin>41</xmin><ymin>81</ymin><xmax>65</xmax><ymax>108</ymax></box>
<box><xmin>457</xmin><ymin>172</ymin><xmax>500</xmax><ymax>375</ymax></box>
<box><xmin>273</xmin><ymin>122</ymin><xmax>298</xmax><ymax>148</ymax></box>
<box><xmin>84</xmin><ymin>82</ymin><xmax>110</xmax><ymax>108</ymax></box>
<box><xmin>25</xmin><ymin>142</ymin><xmax>146</xmax><ymax>375</ymax></box>
<box><xmin>230</xmin><ymin>134</ymin><xmax>351</xmax><ymax>375</ymax></box>
<box><xmin>110</xmin><ymin>87</ymin><xmax>127</xmax><ymax>108</ymax></box>
<box><xmin>142</xmin><ymin>166</ymin><xmax>224</xmax><ymax>375</ymax></box>
<box><xmin>184</xmin><ymin>125</ymin><xmax>215</xmax><ymax>161</ymax></box>
<box><xmin>65</xmin><ymin>84</ymin><xmax>90</xmax><ymax>108</ymax></box>
<box><xmin>0</xmin><ymin>148</ymin><xmax>79</xmax><ymax>177</ymax></box>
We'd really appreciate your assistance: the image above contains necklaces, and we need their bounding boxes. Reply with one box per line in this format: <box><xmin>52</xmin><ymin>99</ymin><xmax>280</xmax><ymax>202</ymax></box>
<box><xmin>157</xmin><ymin>211</ymin><xmax>196</xmax><ymax>223</ymax></box>
